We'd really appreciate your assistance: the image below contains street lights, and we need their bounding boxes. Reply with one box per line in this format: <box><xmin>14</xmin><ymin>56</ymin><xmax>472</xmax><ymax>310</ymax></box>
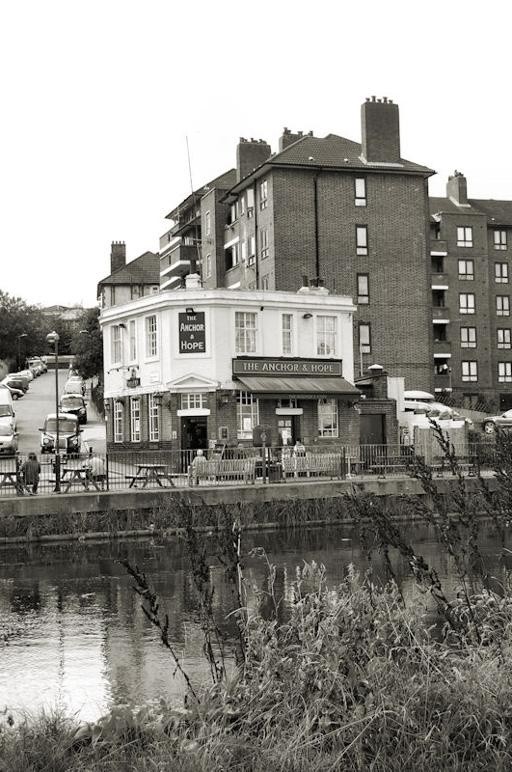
<box><xmin>45</xmin><ymin>330</ymin><xmax>61</xmax><ymax>493</ymax></box>
<box><xmin>80</xmin><ymin>329</ymin><xmax>93</xmax><ymax>400</ymax></box>
<box><xmin>17</xmin><ymin>334</ymin><xmax>28</xmax><ymax>368</ymax></box>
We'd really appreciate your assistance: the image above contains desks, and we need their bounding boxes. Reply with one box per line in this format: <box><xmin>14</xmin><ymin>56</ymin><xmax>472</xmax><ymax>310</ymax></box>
<box><xmin>0</xmin><ymin>462</ymin><xmax>183</xmax><ymax>493</ymax></box>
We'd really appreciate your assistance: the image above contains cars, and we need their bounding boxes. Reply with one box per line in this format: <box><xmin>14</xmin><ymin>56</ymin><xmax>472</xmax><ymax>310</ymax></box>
<box><xmin>0</xmin><ymin>360</ymin><xmax>87</xmax><ymax>460</ymax></box>
<box><xmin>481</xmin><ymin>408</ymin><xmax>512</xmax><ymax>434</ymax></box>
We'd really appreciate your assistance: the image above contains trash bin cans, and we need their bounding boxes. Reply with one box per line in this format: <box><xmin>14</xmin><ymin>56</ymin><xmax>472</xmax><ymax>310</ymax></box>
<box><xmin>269</xmin><ymin>464</ymin><xmax>282</xmax><ymax>484</ymax></box>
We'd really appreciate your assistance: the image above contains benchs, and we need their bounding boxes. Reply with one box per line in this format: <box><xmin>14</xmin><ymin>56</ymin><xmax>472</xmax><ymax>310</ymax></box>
<box><xmin>186</xmin><ymin>453</ymin><xmax>342</xmax><ymax>487</ymax></box>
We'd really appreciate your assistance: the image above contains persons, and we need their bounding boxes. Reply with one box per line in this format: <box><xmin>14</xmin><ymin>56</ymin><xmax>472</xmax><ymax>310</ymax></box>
<box><xmin>191</xmin><ymin>449</ymin><xmax>207</xmax><ymax>473</ymax></box>
<box><xmin>282</xmin><ymin>438</ymin><xmax>306</xmax><ymax>457</ymax></box>
<box><xmin>21</xmin><ymin>452</ymin><xmax>41</xmax><ymax>496</ymax></box>
<box><xmin>82</xmin><ymin>457</ymin><xmax>105</xmax><ymax>492</ymax></box>
